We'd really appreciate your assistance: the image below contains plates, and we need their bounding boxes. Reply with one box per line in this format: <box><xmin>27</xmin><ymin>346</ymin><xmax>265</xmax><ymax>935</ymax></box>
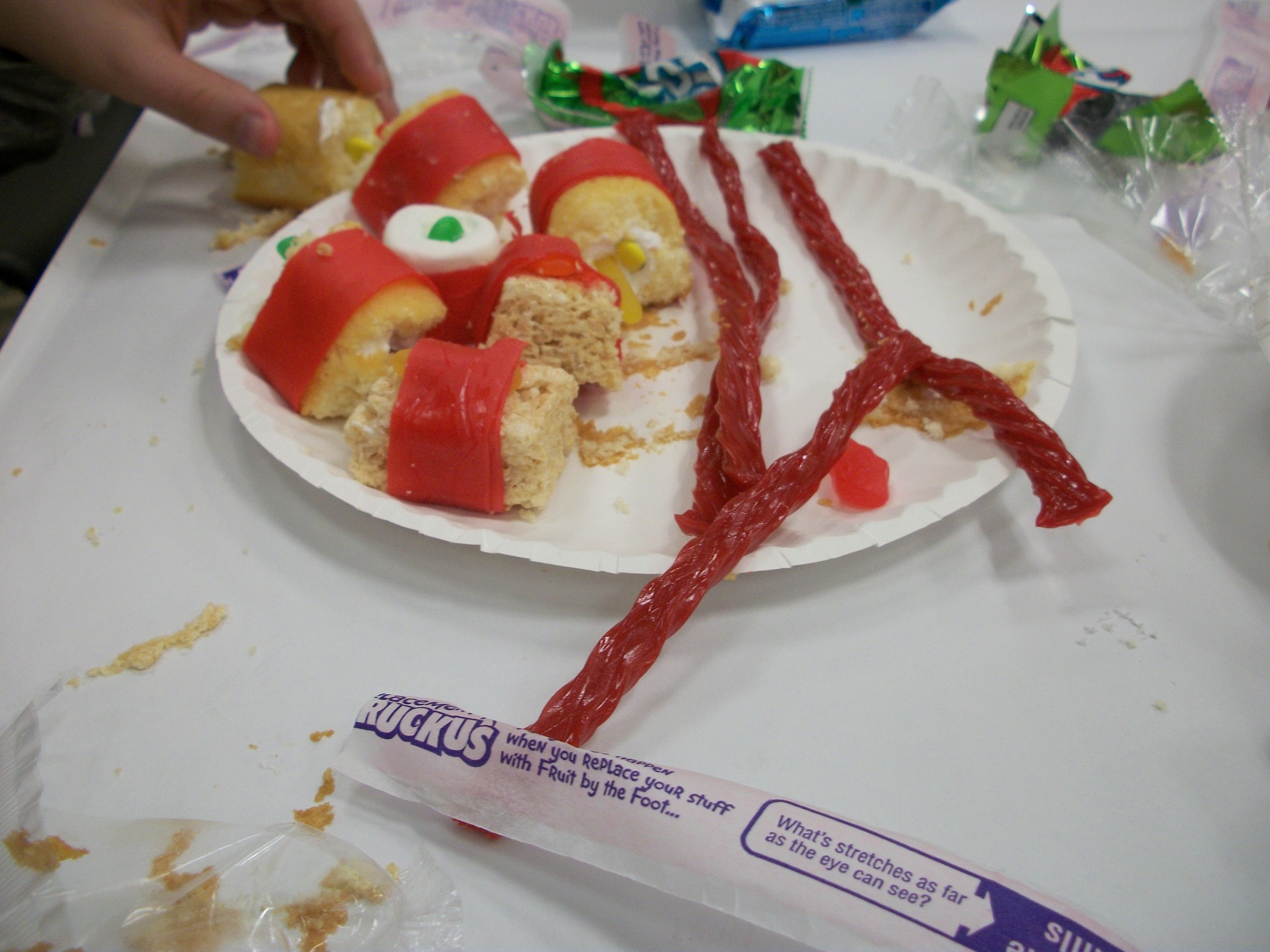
<box><xmin>214</xmin><ymin>124</ymin><xmax>1077</xmax><ymax>575</ymax></box>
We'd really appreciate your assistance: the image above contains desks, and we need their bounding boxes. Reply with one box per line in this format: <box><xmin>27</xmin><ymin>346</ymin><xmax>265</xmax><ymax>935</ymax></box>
<box><xmin>0</xmin><ymin>0</ymin><xmax>1270</xmax><ymax>952</ymax></box>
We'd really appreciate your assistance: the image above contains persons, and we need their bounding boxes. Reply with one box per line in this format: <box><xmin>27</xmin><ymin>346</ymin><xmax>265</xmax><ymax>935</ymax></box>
<box><xmin>0</xmin><ymin>0</ymin><xmax>401</xmax><ymax>160</ymax></box>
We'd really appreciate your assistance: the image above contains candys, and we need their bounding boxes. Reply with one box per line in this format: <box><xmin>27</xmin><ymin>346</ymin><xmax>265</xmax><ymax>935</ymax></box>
<box><xmin>828</xmin><ymin>437</ymin><xmax>889</xmax><ymax>507</ymax></box>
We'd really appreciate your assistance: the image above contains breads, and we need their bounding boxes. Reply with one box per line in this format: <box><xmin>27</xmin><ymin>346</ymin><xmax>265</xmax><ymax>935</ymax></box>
<box><xmin>227</xmin><ymin>81</ymin><xmax>694</xmax><ymax>514</ymax></box>
